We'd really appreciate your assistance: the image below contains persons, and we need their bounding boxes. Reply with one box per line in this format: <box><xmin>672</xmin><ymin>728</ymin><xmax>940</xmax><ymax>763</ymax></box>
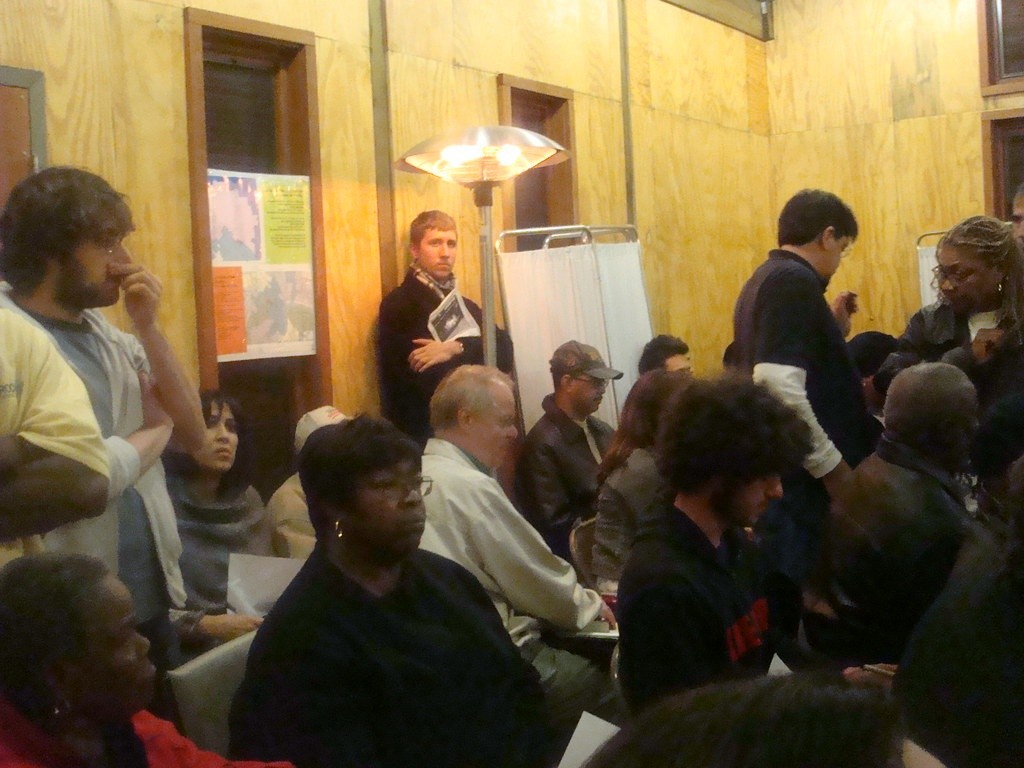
<box><xmin>0</xmin><ymin>164</ymin><xmax>207</xmax><ymax>733</ymax></box>
<box><xmin>592</xmin><ymin>368</ymin><xmax>899</xmax><ymax>715</ymax></box>
<box><xmin>723</xmin><ymin>188</ymin><xmax>885</xmax><ymax>653</ymax></box>
<box><xmin>416</xmin><ymin>363</ymin><xmax>625</xmax><ymax>768</ymax></box>
<box><xmin>637</xmin><ymin>332</ymin><xmax>692</xmax><ymax>376</ymax></box>
<box><xmin>0</xmin><ymin>550</ymin><xmax>297</xmax><ymax>768</ymax></box>
<box><xmin>227</xmin><ymin>408</ymin><xmax>550</xmax><ymax>768</ymax></box>
<box><xmin>264</xmin><ymin>406</ymin><xmax>352</xmax><ymax>560</ymax></box>
<box><xmin>377</xmin><ymin>211</ymin><xmax>514</xmax><ymax>448</ymax></box>
<box><xmin>579</xmin><ymin>676</ymin><xmax>948</xmax><ymax>768</ymax></box>
<box><xmin>514</xmin><ymin>339</ymin><xmax>623</xmax><ymax>567</ymax></box>
<box><xmin>166</xmin><ymin>387</ymin><xmax>290</xmax><ymax>663</ymax></box>
<box><xmin>847</xmin><ymin>213</ymin><xmax>1024</xmax><ymax>680</ymax></box>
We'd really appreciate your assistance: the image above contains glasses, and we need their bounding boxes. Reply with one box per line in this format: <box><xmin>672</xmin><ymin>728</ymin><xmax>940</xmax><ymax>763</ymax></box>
<box><xmin>355</xmin><ymin>470</ymin><xmax>434</xmax><ymax>499</ymax></box>
<box><xmin>933</xmin><ymin>265</ymin><xmax>997</xmax><ymax>286</ymax></box>
<box><xmin>466</xmin><ymin>411</ymin><xmax>514</xmax><ymax>429</ymax></box>
<box><xmin>573</xmin><ymin>374</ymin><xmax>608</xmax><ymax>390</ymax></box>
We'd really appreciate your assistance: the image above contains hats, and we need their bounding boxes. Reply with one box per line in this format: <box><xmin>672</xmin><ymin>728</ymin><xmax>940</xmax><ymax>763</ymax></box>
<box><xmin>551</xmin><ymin>341</ymin><xmax>624</xmax><ymax>380</ymax></box>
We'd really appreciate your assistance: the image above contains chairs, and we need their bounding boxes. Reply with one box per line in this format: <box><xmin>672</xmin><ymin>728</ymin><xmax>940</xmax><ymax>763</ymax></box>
<box><xmin>567</xmin><ymin>516</ymin><xmax>595</xmax><ymax>587</ymax></box>
<box><xmin>163</xmin><ymin>628</ymin><xmax>258</xmax><ymax>762</ymax></box>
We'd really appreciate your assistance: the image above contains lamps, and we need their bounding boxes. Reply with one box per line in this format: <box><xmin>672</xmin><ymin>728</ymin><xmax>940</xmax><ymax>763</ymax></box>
<box><xmin>398</xmin><ymin>125</ymin><xmax>572</xmax><ymax>369</ymax></box>
